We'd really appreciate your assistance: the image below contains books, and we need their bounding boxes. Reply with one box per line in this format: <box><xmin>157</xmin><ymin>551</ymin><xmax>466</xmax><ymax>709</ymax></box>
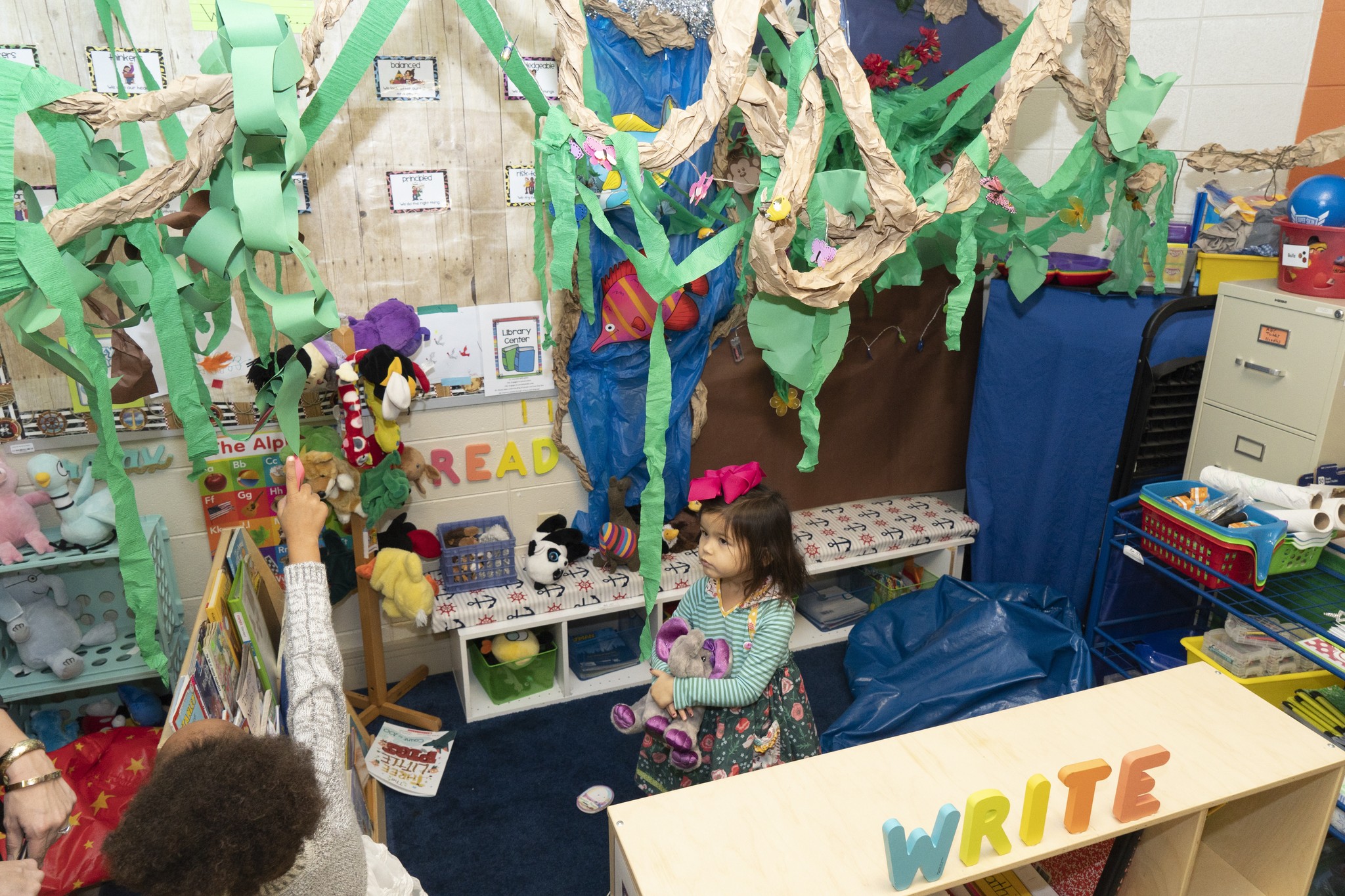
<box><xmin>167</xmin><ymin>527</ymin><xmax>373</xmax><ymax>838</ymax></box>
<box><xmin>937</xmin><ymin>829</ymin><xmax>1149</xmax><ymax>896</ymax></box>
<box><xmin>364</xmin><ymin>722</ymin><xmax>457</xmax><ymax>797</ymax></box>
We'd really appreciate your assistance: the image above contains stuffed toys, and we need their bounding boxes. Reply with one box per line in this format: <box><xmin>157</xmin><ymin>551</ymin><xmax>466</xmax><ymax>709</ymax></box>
<box><xmin>248</xmin><ymin>299</ymin><xmax>442</xmax><ymax>536</ymax></box>
<box><xmin>0</xmin><ymin>454</ymin><xmax>167</xmax><ymax>751</ymax></box>
<box><xmin>443</xmin><ymin>524</ymin><xmax>510</xmax><ymax>582</ymax></box>
<box><xmin>610</xmin><ymin>615</ymin><xmax>733</xmax><ymax>771</ymax></box>
<box><xmin>593</xmin><ymin>477</ymin><xmax>641</xmax><ymax>572</ymax></box>
<box><xmin>670</xmin><ymin>496</ymin><xmax>715</xmax><ymax>551</ymax></box>
<box><xmin>480</xmin><ymin>628</ymin><xmax>540</xmax><ymax>670</ymax></box>
<box><xmin>352</xmin><ymin>512</ymin><xmax>441</xmax><ymax>627</ymax></box>
<box><xmin>524</xmin><ymin>514</ymin><xmax>590</xmax><ymax>590</ymax></box>
<box><xmin>661</xmin><ymin>522</ymin><xmax>688</xmax><ymax>553</ymax></box>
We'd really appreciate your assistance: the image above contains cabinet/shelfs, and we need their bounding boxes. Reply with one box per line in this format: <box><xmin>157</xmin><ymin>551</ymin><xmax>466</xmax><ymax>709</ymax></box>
<box><xmin>606</xmin><ymin>660</ymin><xmax>1345</xmax><ymax>896</ymax></box>
<box><xmin>1085</xmin><ymin>493</ymin><xmax>1345</xmax><ymax>842</ymax></box>
<box><xmin>0</xmin><ymin>514</ymin><xmax>190</xmax><ymax>733</ymax></box>
<box><xmin>1182</xmin><ymin>278</ymin><xmax>1345</xmax><ymax>485</ymax></box>
<box><xmin>448</xmin><ymin>537</ymin><xmax>975</xmax><ymax>724</ymax></box>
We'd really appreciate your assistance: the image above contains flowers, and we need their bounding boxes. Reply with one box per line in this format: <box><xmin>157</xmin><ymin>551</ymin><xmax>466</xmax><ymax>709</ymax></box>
<box><xmin>861</xmin><ymin>26</ymin><xmax>969</xmax><ymax>106</ymax></box>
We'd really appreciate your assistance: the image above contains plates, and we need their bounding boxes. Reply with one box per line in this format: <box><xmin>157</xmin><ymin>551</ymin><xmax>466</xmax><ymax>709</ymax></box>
<box><xmin>992</xmin><ymin>252</ymin><xmax>1115</xmax><ymax>286</ymax></box>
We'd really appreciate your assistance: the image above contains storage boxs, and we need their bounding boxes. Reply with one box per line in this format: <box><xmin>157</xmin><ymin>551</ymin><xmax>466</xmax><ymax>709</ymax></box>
<box><xmin>1141</xmin><ymin>223</ymin><xmax>1191</xmax><ymax>289</ymax></box>
<box><xmin>436</xmin><ymin>515</ymin><xmax>520</xmax><ymax>594</ymax></box>
<box><xmin>1180</xmin><ymin>624</ymin><xmax>1345</xmax><ymax>710</ymax></box>
<box><xmin>468</xmin><ymin>640</ymin><xmax>557</xmax><ymax>706</ymax></box>
<box><xmin>1197</xmin><ymin>251</ymin><xmax>1279</xmax><ymax>295</ymax></box>
<box><xmin>568</xmin><ymin>609</ymin><xmax>645</xmax><ymax>681</ymax></box>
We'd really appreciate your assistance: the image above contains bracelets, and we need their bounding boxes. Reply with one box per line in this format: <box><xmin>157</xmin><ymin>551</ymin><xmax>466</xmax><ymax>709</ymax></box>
<box><xmin>5</xmin><ymin>770</ymin><xmax>62</xmax><ymax>793</ymax></box>
<box><xmin>0</xmin><ymin>739</ymin><xmax>46</xmax><ymax>785</ymax></box>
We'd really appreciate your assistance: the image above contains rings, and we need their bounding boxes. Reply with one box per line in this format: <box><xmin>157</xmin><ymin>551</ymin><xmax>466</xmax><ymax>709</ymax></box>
<box><xmin>61</xmin><ymin>823</ymin><xmax>71</xmax><ymax>835</ymax></box>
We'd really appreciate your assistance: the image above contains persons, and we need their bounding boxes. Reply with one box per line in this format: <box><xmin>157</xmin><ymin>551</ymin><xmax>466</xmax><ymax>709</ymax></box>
<box><xmin>0</xmin><ymin>694</ymin><xmax>76</xmax><ymax>896</ymax></box>
<box><xmin>98</xmin><ymin>455</ymin><xmax>429</xmax><ymax>896</ymax></box>
<box><xmin>634</xmin><ymin>463</ymin><xmax>820</xmax><ymax>794</ymax></box>
